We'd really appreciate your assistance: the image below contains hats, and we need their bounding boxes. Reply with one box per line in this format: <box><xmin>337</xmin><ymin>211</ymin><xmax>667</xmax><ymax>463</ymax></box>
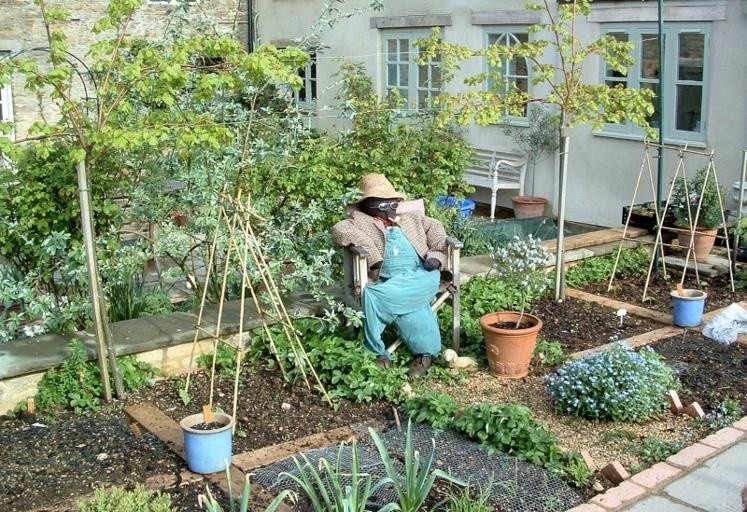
<box><xmin>348</xmin><ymin>173</ymin><xmax>406</xmax><ymax>205</ymax></box>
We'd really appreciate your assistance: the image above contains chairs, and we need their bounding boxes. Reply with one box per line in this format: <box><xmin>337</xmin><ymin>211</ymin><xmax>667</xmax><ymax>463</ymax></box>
<box><xmin>343</xmin><ymin>199</ymin><xmax>464</xmax><ymax>355</ymax></box>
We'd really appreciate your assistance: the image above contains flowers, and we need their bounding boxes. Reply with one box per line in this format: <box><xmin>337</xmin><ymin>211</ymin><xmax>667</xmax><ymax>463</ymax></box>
<box><xmin>488</xmin><ymin>233</ymin><xmax>556</xmax><ymax>327</ymax></box>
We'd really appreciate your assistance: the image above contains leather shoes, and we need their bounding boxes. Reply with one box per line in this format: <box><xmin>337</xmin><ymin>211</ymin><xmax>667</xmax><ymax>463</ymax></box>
<box><xmin>408</xmin><ymin>355</ymin><xmax>432</xmax><ymax>376</ymax></box>
<box><xmin>375</xmin><ymin>357</ymin><xmax>391</xmax><ymax>369</ymax></box>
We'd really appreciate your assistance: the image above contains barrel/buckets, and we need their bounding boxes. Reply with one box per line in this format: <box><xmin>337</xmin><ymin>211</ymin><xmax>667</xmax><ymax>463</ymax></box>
<box><xmin>436</xmin><ymin>195</ymin><xmax>476</xmax><ymax>219</ymax></box>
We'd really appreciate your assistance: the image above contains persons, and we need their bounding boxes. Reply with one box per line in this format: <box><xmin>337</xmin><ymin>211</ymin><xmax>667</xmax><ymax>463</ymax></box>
<box><xmin>330</xmin><ymin>172</ymin><xmax>452</xmax><ymax>381</ymax></box>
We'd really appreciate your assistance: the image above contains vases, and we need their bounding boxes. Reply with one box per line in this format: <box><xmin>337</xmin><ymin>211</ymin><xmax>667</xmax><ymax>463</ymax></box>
<box><xmin>480</xmin><ymin>311</ymin><xmax>543</xmax><ymax>380</ymax></box>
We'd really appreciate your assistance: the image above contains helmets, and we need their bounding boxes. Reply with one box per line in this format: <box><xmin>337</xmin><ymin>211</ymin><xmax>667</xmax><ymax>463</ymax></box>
<box><xmin>368</xmin><ymin>202</ymin><xmax>401</xmax><ymax>210</ymax></box>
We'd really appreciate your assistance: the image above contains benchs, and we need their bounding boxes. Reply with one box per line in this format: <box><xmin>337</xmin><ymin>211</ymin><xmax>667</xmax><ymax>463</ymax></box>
<box><xmin>462</xmin><ymin>143</ymin><xmax>529</xmax><ymax>222</ymax></box>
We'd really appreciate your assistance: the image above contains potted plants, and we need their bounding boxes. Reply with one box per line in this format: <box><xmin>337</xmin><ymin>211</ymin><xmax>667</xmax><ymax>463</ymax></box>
<box><xmin>498</xmin><ymin>104</ymin><xmax>560</xmax><ymax>219</ymax></box>
<box><xmin>670</xmin><ymin>165</ymin><xmax>728</xmax><ymax>258</ymax></box>
